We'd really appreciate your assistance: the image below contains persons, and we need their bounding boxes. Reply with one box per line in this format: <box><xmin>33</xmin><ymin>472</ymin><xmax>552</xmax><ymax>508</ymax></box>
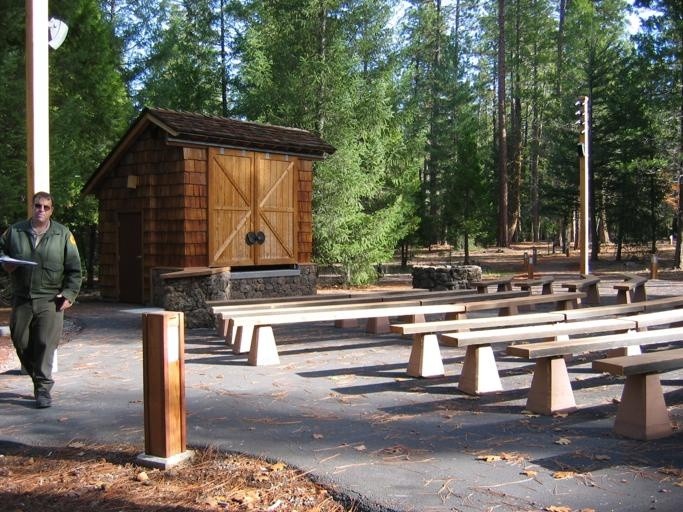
<box><xmin>0</xmin><ymin>191</ymin><xmax>82</xmax><ymax>409</ymax></box>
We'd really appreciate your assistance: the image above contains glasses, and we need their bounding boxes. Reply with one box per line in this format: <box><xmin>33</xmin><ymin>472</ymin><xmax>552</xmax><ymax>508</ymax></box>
<box><xmin>34</xmin><ymin>204</ymin><xmax>51</xmax><ymax>211</ymax></box>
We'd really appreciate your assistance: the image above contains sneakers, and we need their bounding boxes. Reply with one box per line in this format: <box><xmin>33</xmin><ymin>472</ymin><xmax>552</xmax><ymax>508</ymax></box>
<box><xmin>37</xmin><ymin>391</ymin><xmax>51</xmax><ymax>408</ymax></box>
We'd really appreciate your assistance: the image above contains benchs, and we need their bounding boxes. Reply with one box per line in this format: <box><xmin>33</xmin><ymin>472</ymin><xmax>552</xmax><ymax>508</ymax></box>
<box><xmin>199</xmin><ymin>273</ymin><xmax>464</xmax><ymax>366</ymax></box>
<box><xmin>391</xmin><ymin>273</ymin><xmax>682</xmax><ymax>440</ymax></box>
